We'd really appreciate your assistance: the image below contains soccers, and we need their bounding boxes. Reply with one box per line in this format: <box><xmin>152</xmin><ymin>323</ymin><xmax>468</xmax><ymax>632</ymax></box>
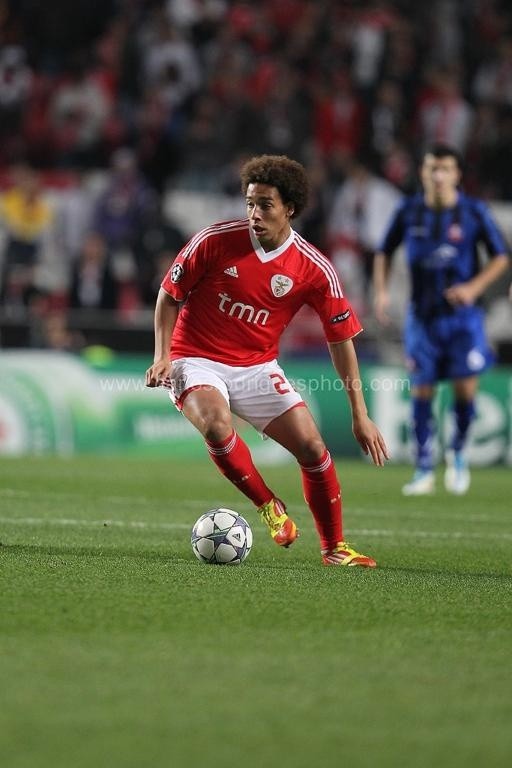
<box><xmin>190</xmin><ymin>509</ymin><xmax>252</xmax><ymax>563</ymax></box>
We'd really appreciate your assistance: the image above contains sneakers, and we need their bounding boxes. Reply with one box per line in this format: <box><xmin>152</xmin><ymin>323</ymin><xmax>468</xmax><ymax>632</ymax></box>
<box><xmin>444</xmin><ymin>447</ymin><xmax>470</xmax><ymax>496</ymax></box>
<box><xmin>320</xmin><ymin>541</ymin><xmax>377</xmax><ymax>568</ymax></box>
<box><xmin>400</xmin><ymin>470</ymin><xmax>434</xmax><ymax>497</ymax></box>
<box><xmin>258</xmin><ymin>495</ymin><xmax>300</xmax><ymax>549</ymax></box>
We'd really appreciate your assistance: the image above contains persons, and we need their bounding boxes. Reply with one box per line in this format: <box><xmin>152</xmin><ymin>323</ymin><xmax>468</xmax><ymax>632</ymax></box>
<box><xmin>371</xmin><ymin>139</ymin><xmax>509</xmax><ymax>503</ymax></box>
<box><xmin>0</xmin><ymin>0</ymin><xmax>510</xmax><ymax>375</ymax></box>
<box><xmin>141</xmin><ymin>152</ymin><xmax>389</xmax><ymax>571</ymax></box>
